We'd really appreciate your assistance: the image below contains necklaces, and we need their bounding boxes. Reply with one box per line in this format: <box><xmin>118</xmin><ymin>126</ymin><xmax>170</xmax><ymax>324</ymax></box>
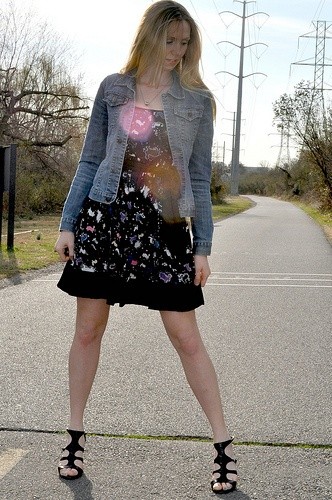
<box><xmin>137</xmin><ymin>77</ymin><xmax>174</xmax><ymax>106</ymax></box>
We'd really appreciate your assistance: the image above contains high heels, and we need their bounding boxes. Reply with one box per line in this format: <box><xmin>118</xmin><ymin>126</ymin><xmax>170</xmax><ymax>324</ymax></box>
<box><xmin>211</xmin><ymin>437</ymin><xmax>238</xmax><ymax>494</ymax></box>
<box><xmin>57</xmin><ymin>429</ymin><xmax>87</xmax><ymax>480</ymax></box>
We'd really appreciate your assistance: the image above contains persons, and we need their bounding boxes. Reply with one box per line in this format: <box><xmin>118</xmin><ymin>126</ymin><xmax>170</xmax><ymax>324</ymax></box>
<box><xmin>54</xmin><ymin>0</ymin><xmax>239</xmax><ymax>493</ymax></box>
